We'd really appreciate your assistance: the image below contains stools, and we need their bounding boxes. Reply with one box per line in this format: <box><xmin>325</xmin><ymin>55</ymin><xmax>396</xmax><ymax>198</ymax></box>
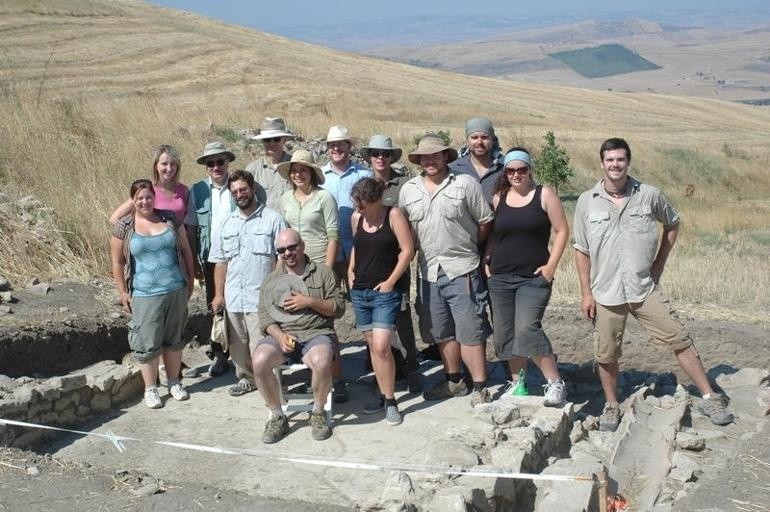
<box><xmin>269</xmin><ymin>362</ymin><xmax>333</xmax><ymax>428</ymax></box>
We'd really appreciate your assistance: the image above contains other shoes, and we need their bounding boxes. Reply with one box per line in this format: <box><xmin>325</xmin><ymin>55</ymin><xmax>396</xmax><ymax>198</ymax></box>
<box><xmin>158</xmin><ymin>365</ymin><xmax>169</xmax><ymax>387</ymax></box>
<box><xmin>208</xmin><ymin>354</ymin><xmax>229</xmax><ymax>377</ymax></box>
<box><xmin>333</xmin><ymin>381</ymin><xmax>348</xmax><ymax>403</ymax></box>
<box><xmin>365</xmin><ymin>345</ymin><xmax>442</xmax><ymax>396</ymax></box>
<box><xmin>178</xmin><ymin>362</ymin><xmax>198</xmax><ymax>378</ymax></box>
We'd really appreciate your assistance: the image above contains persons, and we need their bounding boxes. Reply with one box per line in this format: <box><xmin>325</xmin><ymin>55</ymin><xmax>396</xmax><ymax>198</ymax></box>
<box><xmin>109</xmin><ymin>116</ymin><xmax>568</xmax><ymax>443</ymax></box>
<box><xmin>572</xmin><ymin>138</ymin><xmax>733</xmax><ymax>431</ymax></box>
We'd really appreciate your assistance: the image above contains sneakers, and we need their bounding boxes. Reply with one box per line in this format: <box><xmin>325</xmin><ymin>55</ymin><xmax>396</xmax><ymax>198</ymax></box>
<box><xmin>541</xmin><ymin>379</ymin><xmax>567</xmax><ymax>407</ymax></box>
<box><xmin>167</xmin><ymin>382</ymin><xmax>189</xmax><ymax>401</ymax></box>
<box><xmin>498</xmin><ymin>380</ymin><xmax>528</xmax><ymax>401</ymax></box>
<box><xmin>362</xmin><ymin>398</ymin><xmax>385</xmax><ymax>415</ymax></box>
<box><xmin>698</xmin><ymin>397</ymin><xmax>733</xmax><ymax>425</ymax></box>
<box><xmin>311</xmin><ymin>410</ymin><xmax>331</xmax><ymax>441</ymax></box>
<box><xmin>143</xmin><ymin>386</ymin><xmax>163</xmax><ymax>409</ymax></box>
<box><xmin>423</xmin><ymin>376</ymin><xmax>469</xmax><ymax>400</ymax></box>
<box><xmin>262</xmin><ymin>415</ymin><xmax>289</xmax><ymax>443</ymax></box>
<box><xmin>469</xmin><ymin>387</ymin><xmax>491</xmax><ymax>408</ymax></box>
<box><xmin>599</xmin><ymin>401</ymin><xmax>621</xmax><ymax>431</ymax></box>
<box><xmin>229</xmin><ymin>378</ymin><xmax>257</xmax><ymax>396</ymax></box>
<box><xmin>384</xmin><ymin>402</ymin><xmax>402</xmax><ymax>426</ymax></box>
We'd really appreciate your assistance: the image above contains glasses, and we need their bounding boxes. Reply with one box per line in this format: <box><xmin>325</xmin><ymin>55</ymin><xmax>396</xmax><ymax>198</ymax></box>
<box><xmin>206</xmin><ymin>158</ymin><xmax>228</xmax><ymax>167</ymax></box>
<box><xmin>369</xmin><ymin>151</ymin><xmax>390</xmax><ymax>158</ymax></box>
<box><xmin>277</xmin><ymin>242</ymin><xmax>299</xmax><ymax>253</ymax></box>
<box><xmin>506</xmin><ymin>167</ymin><xmax>528</xmax><ymax>176</ymax></box>
<box><xmin>263</xmin><ymin>138</ymin><xmax>281</xmax><ymax>142</ymax></box>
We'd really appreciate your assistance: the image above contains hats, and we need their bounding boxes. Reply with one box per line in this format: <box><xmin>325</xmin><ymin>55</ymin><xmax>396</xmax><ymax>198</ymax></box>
<box><xmin>211</xmin><ymin>309</ymin><xmax>229</xmax><ymax>353</ymax></box>
<box><xmin>276</xmin><ymin>150</ymin><xmax>324</xmax><ymax>185</ymax></box>
<box><xmin>263</xmin><ymin>274</ymin><xmax>309</xmax><ymax>323</ymax></box>
<box><xmin>360</xmin><ymin>136</ymin><xmax>401</xmax><ymax>164</ymax></box>
<box><xmin>327</xmin><ymin>125</ymin><xmax>357</xmax><ymax>145</ymax></box>
<box><xmin>197</xmin><ymin>141</ymin><xmax>235</xmax><ymax>165</ymax></box>
<box><xmin>409</xmin><ymin>137</ymin><xmax>457</xmax><ymax>165</ymax></box>
<box><xmin>251</xmin><ymin>117</ymin><xmax>296</xmax><ymax>139</ymax></box>
